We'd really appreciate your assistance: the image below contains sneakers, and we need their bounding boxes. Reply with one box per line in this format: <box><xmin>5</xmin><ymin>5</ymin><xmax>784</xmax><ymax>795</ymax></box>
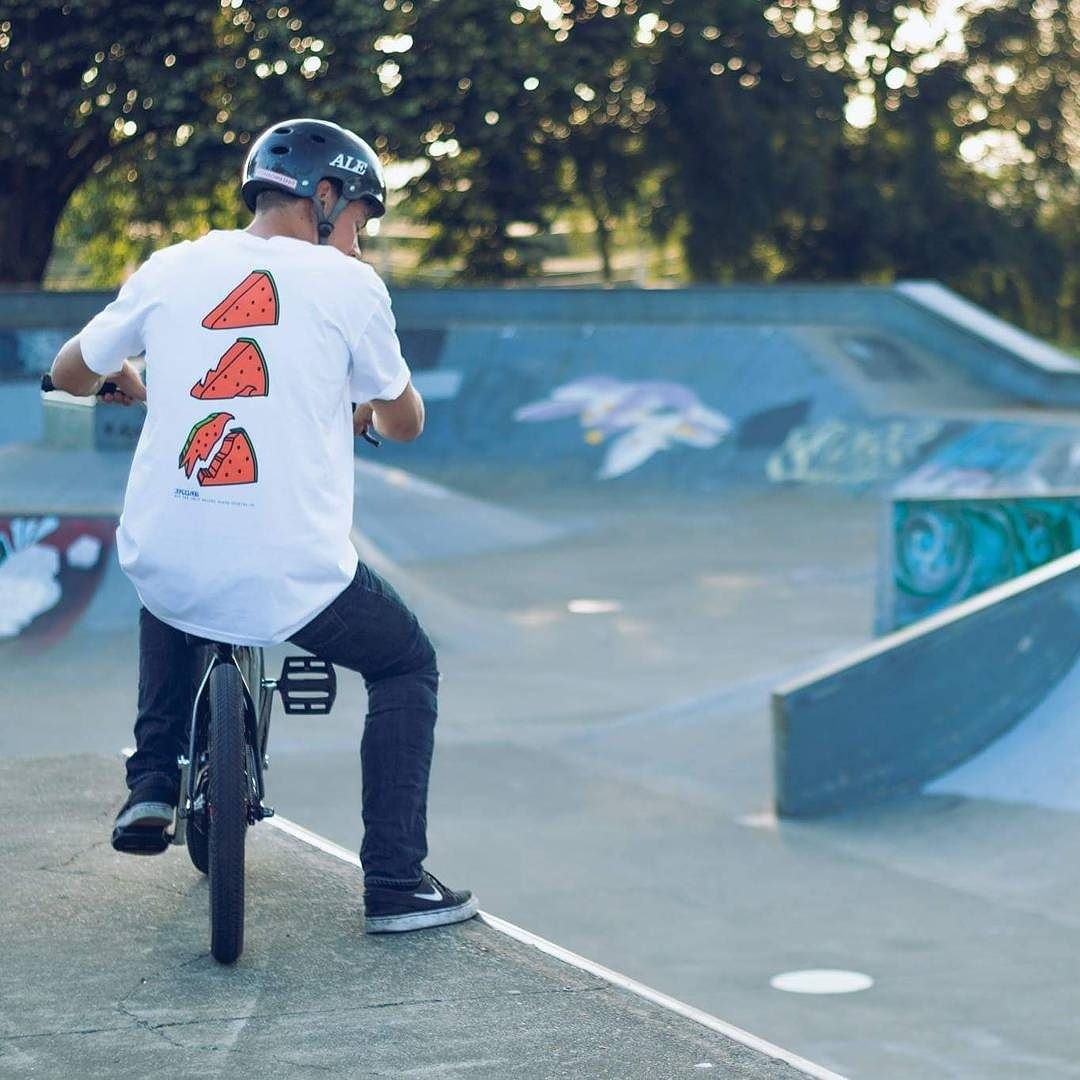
<box><xmin>363</xmin><ymin>865</ymin><xmax>477</xmax><ymax>933</ymax></box>
<box><xmin>109</xmin><ymin>777</ymin><xmax>175</xmax><ymax>856</ymax></box>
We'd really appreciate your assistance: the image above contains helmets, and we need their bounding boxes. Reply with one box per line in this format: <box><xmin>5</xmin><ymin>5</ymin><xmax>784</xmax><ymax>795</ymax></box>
<box><xmin>239</xmin><ymin>118</ymin><xmax>388</xmax><ymax>220</ymax></box>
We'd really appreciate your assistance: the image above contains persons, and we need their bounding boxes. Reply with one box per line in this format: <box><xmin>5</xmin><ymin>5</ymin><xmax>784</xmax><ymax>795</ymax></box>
<box><xmin>51</xmin><ymin>118</ymin><xmax>480</xmax><ymax>933</ymax></box>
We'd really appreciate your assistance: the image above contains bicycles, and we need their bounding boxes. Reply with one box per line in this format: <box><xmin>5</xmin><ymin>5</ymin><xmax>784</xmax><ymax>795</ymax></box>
<box><xmin>40</xmin><ymin>372</ymin><xmax>381</xmax><ymax>963</ymax></box>
